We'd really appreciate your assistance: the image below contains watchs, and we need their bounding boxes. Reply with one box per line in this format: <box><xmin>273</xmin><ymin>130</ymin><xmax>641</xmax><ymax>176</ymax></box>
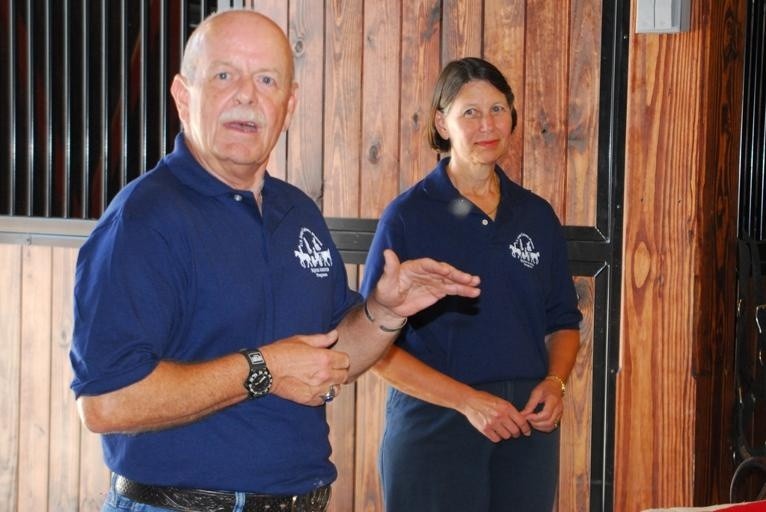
<box><xmin>235</xmin><ymin>346</ymin><xmax>272</xmax><ymax>403</ymax></box>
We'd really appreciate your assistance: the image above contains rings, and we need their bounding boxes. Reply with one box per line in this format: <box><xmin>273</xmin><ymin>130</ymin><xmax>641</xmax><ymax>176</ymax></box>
<box><xmin>321</xmin><ymin>388</ymin><xmax>336</xmax><ymax>405</ymax></box>
<box><xmin>554</xmin><ymin>420</ymin><xmax>560</xmax><ymax>428</ymax></box>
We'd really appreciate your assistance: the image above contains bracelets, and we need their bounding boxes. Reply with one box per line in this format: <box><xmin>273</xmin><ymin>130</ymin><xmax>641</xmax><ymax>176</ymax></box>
<box><xmin>363</xmin><ymin>298</ymin><xmax>408</xmax><ymax>334</ymax></box>
<box><xmin>542</xmin><ymin>373</ymin><xmax>568</xmax><ymax>396</ymax></box>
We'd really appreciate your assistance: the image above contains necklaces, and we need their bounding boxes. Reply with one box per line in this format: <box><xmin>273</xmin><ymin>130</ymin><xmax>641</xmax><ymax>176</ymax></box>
<box><xmin>486</xmin><ymin>208</ymin><xmax>496</xmax><ymax>216</ymax></box>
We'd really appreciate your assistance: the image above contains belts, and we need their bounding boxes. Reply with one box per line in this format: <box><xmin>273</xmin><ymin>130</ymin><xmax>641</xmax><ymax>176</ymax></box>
<box><xmin>106</xmin><ymin>465</ymin><xmax>333</xmax><ymax>512</ymax></box>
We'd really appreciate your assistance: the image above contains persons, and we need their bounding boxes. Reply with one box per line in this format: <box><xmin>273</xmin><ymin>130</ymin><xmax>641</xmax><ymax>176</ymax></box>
<box><xmin>67</xmin><ymin>4</ymin><xmax>482</xmax><ymax>512</ymax></box>
<box><xmin>352</xmin><ymin>55</ymin><xmax>585</xmax><ymax>512</ymax></box>
